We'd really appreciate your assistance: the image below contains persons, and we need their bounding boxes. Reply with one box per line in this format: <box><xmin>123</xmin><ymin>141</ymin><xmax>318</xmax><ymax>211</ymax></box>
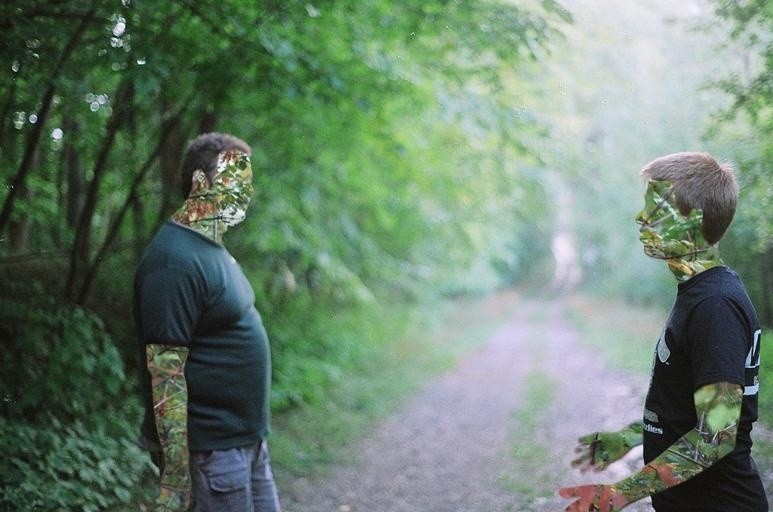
<box><xmin>134</xmin><ymin>131</ymin><xmax>281</xmax><ymax>512</ymax></box>
<box><xmin>559</xmin><ymin>151</ymin><xmax>768</xmax><ymax>512</ymax></box>
